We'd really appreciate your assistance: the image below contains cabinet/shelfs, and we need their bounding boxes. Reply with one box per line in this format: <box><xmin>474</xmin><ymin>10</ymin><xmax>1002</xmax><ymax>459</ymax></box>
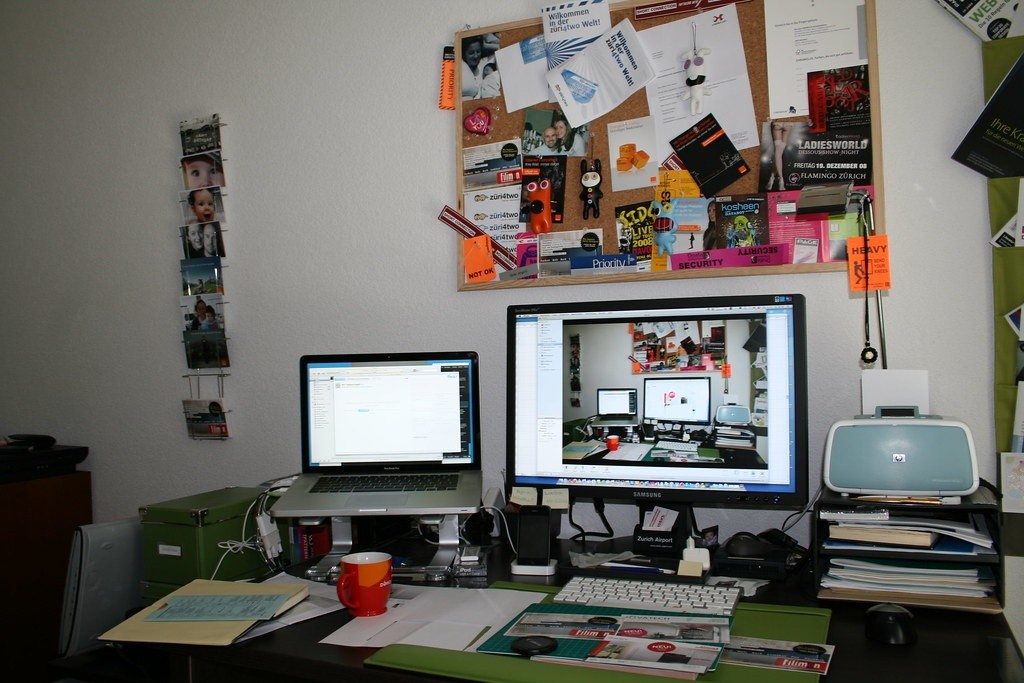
<box><xmin>0</xmin><ymin>471</ymin><xmax>93</xmax><ymax>683</ymax></box>
<box><xmin>981</xmin><ymin>36</ymin><xmax>1023</xmax><ymax>683</ymax></box>
<box><xmin>750</xmin><ymin>320</ymin><xmax>768</xmax><ymax>430</ymax></box>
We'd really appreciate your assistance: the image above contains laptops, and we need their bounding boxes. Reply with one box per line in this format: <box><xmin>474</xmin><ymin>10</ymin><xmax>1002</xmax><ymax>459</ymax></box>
<box><xmin>591</xmin><ymin>388</ymin><xmax>638</xmax><ymax>427</ymax></box>
<box><xmin>270</xmin><ymin>351</ymin><xmax>482</xmax><ymax>517</ymax></box>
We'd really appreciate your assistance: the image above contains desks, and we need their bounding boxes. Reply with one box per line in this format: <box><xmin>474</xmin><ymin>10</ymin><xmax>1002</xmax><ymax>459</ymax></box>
<box><xmin>562</xmin><ymin>431</ymin><xmax>768</xmax><ymax>469</ymax></box>
<box><xmin>125</xmin><ymin>537</ymin><xmax>1023</xmax><ymax>683</ymax></box>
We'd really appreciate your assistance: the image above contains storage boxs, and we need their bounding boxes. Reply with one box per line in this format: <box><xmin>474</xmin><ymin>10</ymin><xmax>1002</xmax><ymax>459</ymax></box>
<box><xmin>562</xmin><ymin>419</ymin><xmax>591</xmax><ymax>442</ymax></box>
<box><xmin>137</xmin><ymin>486</ymin><xmax>292</xmax><ymax>597</ymax></box>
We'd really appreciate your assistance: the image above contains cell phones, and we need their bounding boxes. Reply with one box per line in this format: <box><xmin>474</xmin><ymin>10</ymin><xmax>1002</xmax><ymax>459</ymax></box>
<box><xmin>644</xmin><ymin>424</ymin><xmax>654</xmax><ymax>438</ymax></box>
<box><xmin>517</xmin><ymin>505</ymin><xmax>551</xmax><ymax>566</ymax></box>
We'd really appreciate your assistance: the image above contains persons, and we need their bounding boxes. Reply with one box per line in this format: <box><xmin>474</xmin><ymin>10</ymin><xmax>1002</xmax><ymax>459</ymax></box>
<box><xmin>766</xmin><ymin>120</ymin><xmax>792</xmax><ymax>190</ymax></box>
<box><xmin>181</xmin><ymin>152</ymin><xmax>225</xmax><ymax>365</ymax></box>
<box><xmin>527</xmin><ymin>114</ymin><xmax>585</xmax><ymax>155</ymax></box>
<box><xmin>702</xmin><ymin>199</ymin><xmax>716</xmax><ymax>250</ymax></box>
<box><xmin>461</xmin><ymin>34</ymin><xmax>500</xmax><ymax>100</ymax></box>
<box><xmin>703</xmin><ymin>529</ymin><xmax>717</xmax><ymax>545</ymax></box>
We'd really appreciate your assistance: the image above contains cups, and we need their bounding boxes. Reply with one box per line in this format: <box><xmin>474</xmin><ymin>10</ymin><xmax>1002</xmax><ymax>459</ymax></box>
<box><xmin>336</xmin><ymin>552</ymin><xmax>392</xmax><ymax>619</ymax></box>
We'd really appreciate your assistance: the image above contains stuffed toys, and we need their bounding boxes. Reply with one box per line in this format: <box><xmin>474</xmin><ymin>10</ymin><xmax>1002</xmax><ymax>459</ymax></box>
<box><xmin>652</xmin><ymin>196</ymin><xmax>679</xmax><ymax>256</ymax></box>
<box><xmin>680</xmin><ymin>47</ymin><xmax>712</xmax><ymax>115</ymax></box>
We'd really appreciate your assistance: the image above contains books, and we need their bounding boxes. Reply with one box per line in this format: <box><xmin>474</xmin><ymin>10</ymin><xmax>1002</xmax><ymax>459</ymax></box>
<box><xmin>669</xmin><ymin>427</ymin><xmax>756</xmax><ymax>462</ymax></box>
<box><xmin>562</xmin><ymin>442</ymin><xmax>600</xmax><ymax>460</ymax></box>
<box><xmin>476</xmin><ymin>603</ymin><xmax>730</xmax><ymax>680</ymax></box>
<box><xmin>821</xmin><ymin>519</ymin><xmax>999</xmax><ymax>598</ymax></box>
<box><xmin>97</xmin><ymin>579</ymin><xmax>310</xmax><ymax>646</ymax></box>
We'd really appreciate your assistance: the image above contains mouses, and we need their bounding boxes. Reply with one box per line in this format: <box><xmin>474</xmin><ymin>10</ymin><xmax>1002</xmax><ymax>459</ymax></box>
<box><xmin>865</xmin><ymin>602</ymin><xmax>917</xmax><ymax>652</ymax></box>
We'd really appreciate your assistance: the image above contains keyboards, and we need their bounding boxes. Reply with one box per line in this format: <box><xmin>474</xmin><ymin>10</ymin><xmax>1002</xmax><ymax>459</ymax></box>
<box><xmin>655</xmin><ymin>441</ymin><xmax>697</xmax><ymax>451</ymax></box>
<box><xmin>552</xmin><ymin>577</ymin><xmax>744</xmax><ymax>618</ymax></box>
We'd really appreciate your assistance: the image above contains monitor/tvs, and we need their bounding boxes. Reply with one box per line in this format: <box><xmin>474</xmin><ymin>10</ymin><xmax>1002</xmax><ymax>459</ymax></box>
<box><xmin>507</xmin><ymin>293</ymin><xmax>809</xmax><ymax>561</ymax></box>
<box><xmin>643</xmin><ymin>377</ymin><xmax>711</xmax><ymax>442</ymax></box>
